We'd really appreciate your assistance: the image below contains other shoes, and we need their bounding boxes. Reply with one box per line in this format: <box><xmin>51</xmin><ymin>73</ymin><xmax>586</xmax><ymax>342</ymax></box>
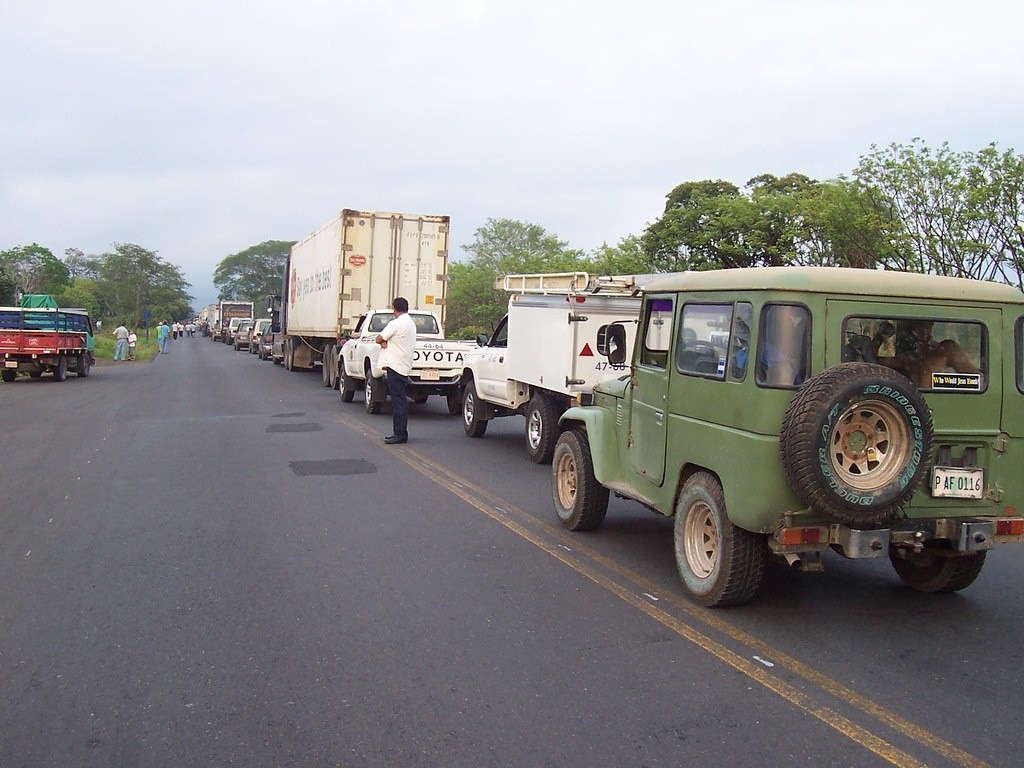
<box><xmin>161</xmin><ymin>351</ymin><xmax>163</xmax><ymax>353</ymax></box>
<box><xmin>165</xmin><ymin>352</ymin><xmax>168</xmax><ymax>354</ymax></box>
<box><xmin>122</xmin><ymin>358</ymin><xmax>125</xmax><ymax>361</ymax></box>
<box><xmin>114</xmin><ymin>357</ymin><xmax>118</xmax><ymax>361</ymax></box>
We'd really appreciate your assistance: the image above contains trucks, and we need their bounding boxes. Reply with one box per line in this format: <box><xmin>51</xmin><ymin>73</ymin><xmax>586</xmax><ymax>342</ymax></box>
<box><xmin>198</xmin><ymin>304</ymin><xmax>219</xmax><ymax>337</ymax></box>
<box><xmin>462</xmin><ymin>270</ymin><xmax>642</xmax><ymax>463</ymax></box>
<box><xmin>217</xmin><ymin>301</ymin><xmax>254</xmax><ymax>342</ymax></box>
<box><xmin>0</xmin><ymin>306</ymin><xmax>97</xmax><ymax>382</ymax></box>
<box><xmin>265</xmin><ymin>208</ymin><xmax>451</xmax><ymax>390</ymax></box>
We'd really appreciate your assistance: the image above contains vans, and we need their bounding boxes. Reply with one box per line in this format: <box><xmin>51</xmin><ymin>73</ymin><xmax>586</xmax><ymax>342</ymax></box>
<box><xmin>226</xmin><ymin>318</ymin><xmax>253</xmax><ymax>345</ymax></box>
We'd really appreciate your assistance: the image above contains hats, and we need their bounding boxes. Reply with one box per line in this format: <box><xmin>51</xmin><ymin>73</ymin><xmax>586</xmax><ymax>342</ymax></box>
<box><xmin>164</xmin><ymin>320</ymin><xmax>167</xmax><ymax>322</ymax></box>
<box><xmin>159</xmin><ymin>322</ymin><xmax>163</xmax><ymax>325</ymax></box>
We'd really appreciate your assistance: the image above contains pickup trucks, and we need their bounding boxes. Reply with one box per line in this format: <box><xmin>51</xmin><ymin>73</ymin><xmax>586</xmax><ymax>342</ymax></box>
<box><xmin>335</xmin><ymin>309</ymin><xmax>486</xmax><ymax>416</ymax></box>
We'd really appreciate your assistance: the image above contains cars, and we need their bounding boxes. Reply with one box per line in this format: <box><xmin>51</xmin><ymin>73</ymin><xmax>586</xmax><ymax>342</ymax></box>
<box><xmin>212</xmin><ymin>325</ymin><xmax>221</xmax><ymax>342</ymax></box>
<box><xmin>232</xmin><ymin>322</ymin><xmax>253</xmax><ymax>351</ymax></box>
<box><xmin>256</xmin><ymin>324</ymin><xmax>273</xmax><ymax>360</ymax></box>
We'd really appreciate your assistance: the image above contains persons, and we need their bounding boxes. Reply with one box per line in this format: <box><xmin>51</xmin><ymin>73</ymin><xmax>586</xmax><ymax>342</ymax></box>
<box><xmin>376</xmin><ymin>297</ymin><xmax>416</xmax><ymax>444</ymax></box>
<box><xmin>156</xmin><ymin>320</ymin><xmax>174</xmax><ymax>354</ymax></box>
<box><xmin>866</xmin><ymin>320</ymin><xmax>983</xmax><ymax>389</ymax></box>
<box><xmin>112</xmin><ymin>322</ymin><xmax>137</xmax><ymax>360</ymax></box>
<box><xmin>352</xmin><ymin>317</ymin><xmax>381</xmax><ymax>338</ymax></box>
<box><xmin>678</xmin><ymin>327</ymin><xmax>696</xmax><ymax>351</ymax></box>
<box><xmin>172</xmin><ymin>321</ymin><xmax>209</xmax><ymax>340</ymax></box>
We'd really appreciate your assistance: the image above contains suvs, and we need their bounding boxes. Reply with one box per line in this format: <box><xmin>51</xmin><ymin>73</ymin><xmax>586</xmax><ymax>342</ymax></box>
<box><xmin>248</xmin><ymin>319</ymin><xmax>273</xmax><ymax>354</ymax></box>
<box><xmin>549</xmin><ymin>265</ymin><xmax>1024</xmax><ymax>611</ymax></box>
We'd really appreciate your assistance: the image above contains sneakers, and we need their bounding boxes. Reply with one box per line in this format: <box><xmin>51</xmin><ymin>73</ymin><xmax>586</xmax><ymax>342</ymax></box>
<box><xmin>127</xmin><ymin>356</ymin><xmax>135</xmax><ymax>361</ymax></box>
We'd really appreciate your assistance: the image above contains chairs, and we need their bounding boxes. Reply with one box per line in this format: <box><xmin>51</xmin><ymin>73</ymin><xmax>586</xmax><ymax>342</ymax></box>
<box><xmin>676</xmin><ymin>327</ymin><xmax>719</xmax><ymax>375</ymax></box>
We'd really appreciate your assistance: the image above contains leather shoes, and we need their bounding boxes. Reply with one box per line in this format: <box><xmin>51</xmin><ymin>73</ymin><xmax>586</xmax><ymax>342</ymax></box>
<box><xmin>385</xmin><ymin>436</ymin><xmax>408</xmax><ymax>444</ymax></box>
